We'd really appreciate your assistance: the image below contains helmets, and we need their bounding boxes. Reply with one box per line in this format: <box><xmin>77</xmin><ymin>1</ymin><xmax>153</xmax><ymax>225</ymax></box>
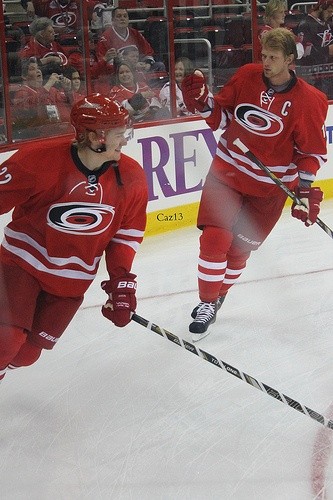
<box><xmin>70</xmin><ymin>92</ymin><xmax>132</xmax><ymax>143</ymax></box>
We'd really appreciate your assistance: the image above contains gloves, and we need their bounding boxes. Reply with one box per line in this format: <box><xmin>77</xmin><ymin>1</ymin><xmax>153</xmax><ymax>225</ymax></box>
<box><xmin>180</xmin><ymin>70</ymin><xmax>209</xmax><ymax>114</ymax></box>
<box><xmin>101</xmin><ymin>273</ymin><xmax>137</xmax><ymax>329</ymax></box>
<box><xmin>290</xmin><ymin>187</ymin><xmax>324</xmax><ymax>225</ymax></box>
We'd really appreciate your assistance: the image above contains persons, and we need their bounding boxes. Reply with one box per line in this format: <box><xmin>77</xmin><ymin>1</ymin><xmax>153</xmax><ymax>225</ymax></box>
<box><xmin>13</xmin><ymin>1</ymin><xmax>333</xmax><ymax>130</ymax></box>
<box><xmin>0</xmin><ymin>93</ymin><xmax>148</xmax><ymax>382</ymax></box>
<box><xmin>181</xmin><ymin>28</ymin><xmax>328</xmax><ymax>342</ymax></box>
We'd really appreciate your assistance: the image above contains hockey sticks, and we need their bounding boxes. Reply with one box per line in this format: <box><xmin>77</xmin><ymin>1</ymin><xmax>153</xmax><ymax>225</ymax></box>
<box><xmin>234</xmin><ymin>137</ymin><xmax>333</xmax><ymax>239</ymax></box>
<box><xmin>103</xmin><ymin>280</ymin><xmax>332</xmax><ymax>429</ymax></box>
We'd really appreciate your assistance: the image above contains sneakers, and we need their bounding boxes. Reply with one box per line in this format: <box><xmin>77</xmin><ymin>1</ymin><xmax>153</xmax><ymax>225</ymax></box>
<box><xmin>190</xmin><ymin>293</ymin><xmax>225</xmax><ymax>318</ymax></box>
<box><xmin>188</xmin><ymin>300</ymin><xmax>216</xmax><ymax>341</ymax></box>
